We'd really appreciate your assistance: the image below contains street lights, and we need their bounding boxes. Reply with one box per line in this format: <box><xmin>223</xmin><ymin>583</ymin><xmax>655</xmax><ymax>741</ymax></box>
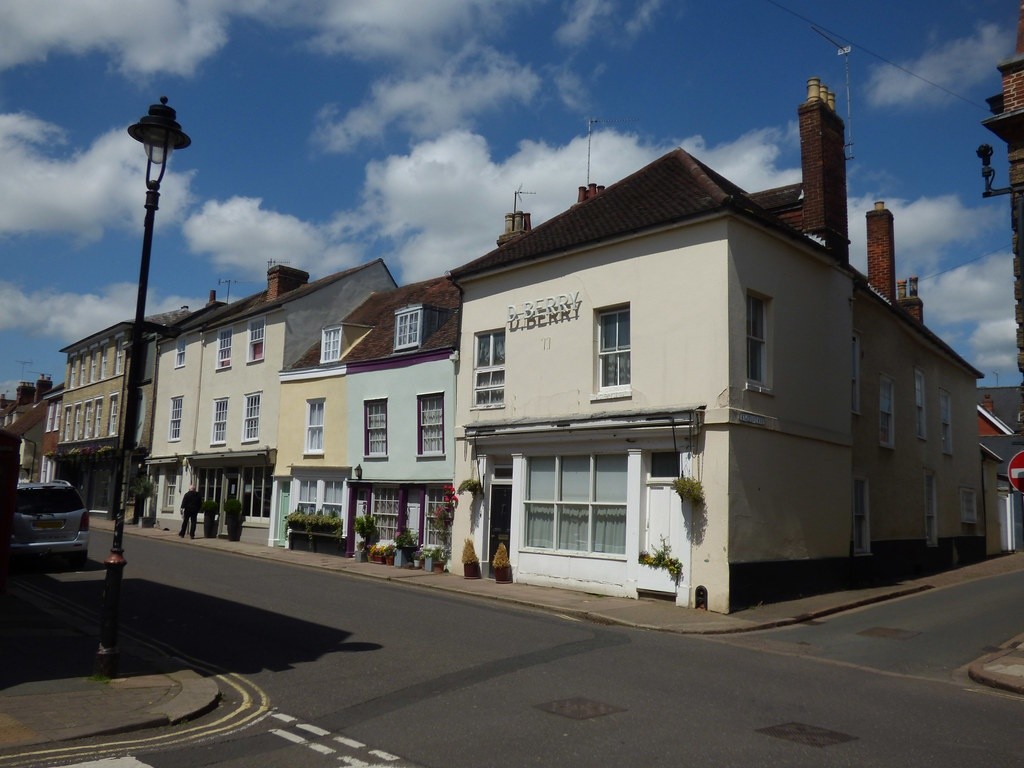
<box><xmin>94</xmin><ymin>97</ymin><xmax>192</xmax><ymax>682</ymax></box>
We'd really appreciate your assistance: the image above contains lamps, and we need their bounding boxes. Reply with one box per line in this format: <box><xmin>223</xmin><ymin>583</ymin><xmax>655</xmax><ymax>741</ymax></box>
<box><xmin>448</xmin><ymin>350</ymin><xmax>460</xmax><ymax>362</ymax></box>
<box><xmin>198</xmin><ymin>323</ymin><xmax>209</xmax><ymax>333</ymax></box>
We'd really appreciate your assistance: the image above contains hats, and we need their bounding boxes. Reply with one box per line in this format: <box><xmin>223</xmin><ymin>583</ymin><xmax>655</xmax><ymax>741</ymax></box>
<box><xmin>189</xmin><ymin>485</ymin><xmax>196</xmax><ymax>492</ymax></box>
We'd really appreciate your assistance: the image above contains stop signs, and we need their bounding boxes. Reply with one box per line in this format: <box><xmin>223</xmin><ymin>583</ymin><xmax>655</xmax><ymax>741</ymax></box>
<box><xmin>1008</xmin><ymin>451</ymin><xmax>1024</xmax><ymax>494</ymax></box>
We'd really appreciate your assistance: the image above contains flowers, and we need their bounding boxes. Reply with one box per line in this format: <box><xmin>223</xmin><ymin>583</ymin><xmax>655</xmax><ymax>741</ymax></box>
<box><xmin>420</xmin><ymin>548</ymin><xmax>434</xmax><ymax>559</ymax></box>
<box><xmin>431</xmin><ymin>485</ymin><xmax>459</xmax><ymax>544</ymax></box>
<box><xmin>44</xmin><ymin>445</ymin><xmax>116</xmax><ymax>463</ymax></box>
<box><xmin>367</xmin><ymin>544</ymin><xmax>395</xmax><ymax>556</ymax></box>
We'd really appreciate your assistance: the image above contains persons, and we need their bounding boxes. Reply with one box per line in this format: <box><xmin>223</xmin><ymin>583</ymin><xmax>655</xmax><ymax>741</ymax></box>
<box><xmin>178</xmin><ymin>485</ymin><xmax>202</xmax><ymax>539</ymax></box>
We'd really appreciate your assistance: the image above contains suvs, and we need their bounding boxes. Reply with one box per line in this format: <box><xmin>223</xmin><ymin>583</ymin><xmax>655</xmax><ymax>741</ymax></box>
<box><xmin>8</xmin><ymin>480</ymin><xmax>89</xmax><ymax>570</ymax></box>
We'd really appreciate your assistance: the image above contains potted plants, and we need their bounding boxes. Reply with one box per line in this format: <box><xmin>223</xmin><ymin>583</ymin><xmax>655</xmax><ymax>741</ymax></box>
<box><xmin>492</xmin><ymin>543</ymin><xmax>513</xmax><ymax>584</ymax></box>
<box><xmin>461</xmin><ymin>538</ymin><xmax>482</xmax><ymax>579</ymax></box>
<box><xmin>637</xmin><ymin>533</ymin><xmax>684</xmax><ymax>592</ymax></box>
<box><xmin>137</xmin><ymin>481</ymin><xmax>155</xmax><ymax>528</ymax></box>
<box><xmin>669</xmin><ymin>476</ymin><xmax>705</xmax><ymax>504</ymax></box>
<box><xmin>200</xmin><ymin>499</ymin><xmax>220</xmax><ymax>537</ymax></box>
<box><xmin>283</xmin><ymin>509</ymin><xmax>343</xmax><ymax>534</ymax></box>
<box><xmin>432</xmin><ymin>546</ymin><xmax>447</xmax><ymax>573</ymax></box>
<box><xmin>394</xmin><ymin>527</ymin><xmax>418</xmax><ymax>567</ymax></box>
<box><xmin>413</xmin><ymin>551</ymin><xmax>420</xmax><ymax>567</ymax></box>
<box><xmin>223</xmin><ymin>498</ymin><xmax>245</xmax><ymax>541</ymax></box>
<box><xmin>457</xmin><ymin>479</ymin><xmax>479</xmax><ymax>496</ymax></box>
<box><xmin>354</xmin><ymin>541</ymin><xmax>368</xmax><ymax>562</ymax></box>
<box><xmin>124</xmin><ymin>485</ymin><xmax>137</xmax><ymax>524</ymax></box>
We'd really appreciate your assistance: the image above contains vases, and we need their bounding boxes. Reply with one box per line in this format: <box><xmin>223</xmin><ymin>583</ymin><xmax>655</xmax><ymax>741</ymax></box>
<box><xmin>425</xmin><ymin>557</ymin><xmax>432</xmax><ymax>572</ymax></box>
<box><xmin>368</xmin><ymin>556</ymin><xmax>394</xmax><ymax>566</ymax></box>
